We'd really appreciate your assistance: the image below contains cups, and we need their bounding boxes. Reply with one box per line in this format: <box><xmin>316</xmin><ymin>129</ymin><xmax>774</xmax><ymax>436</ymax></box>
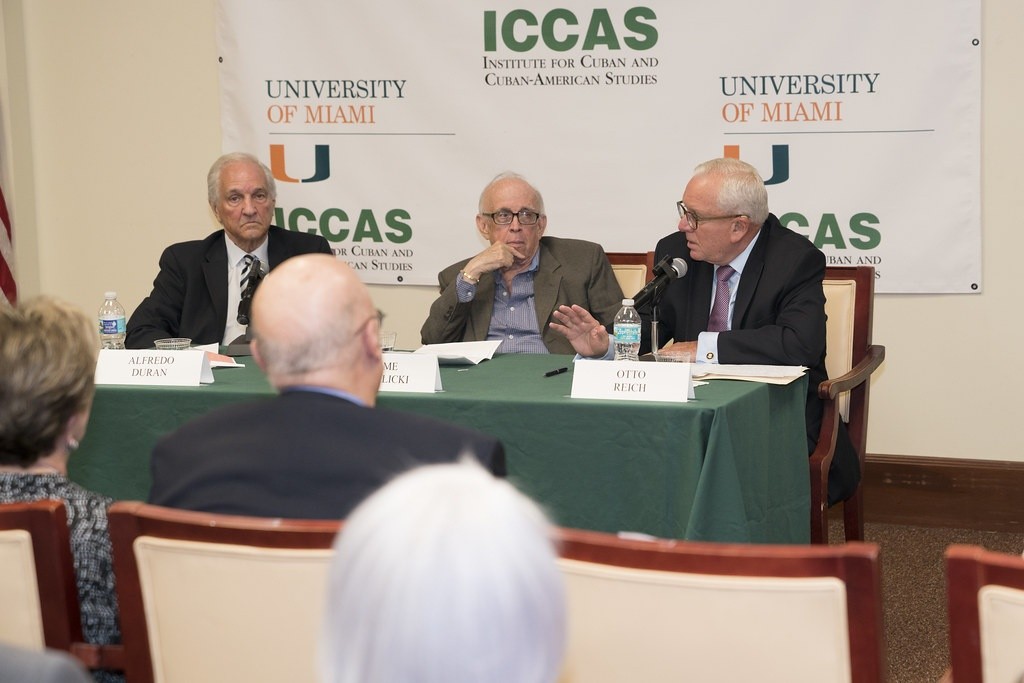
<box><xmin>154</xmin><ymin>338</ymin><xmax>191</xmax><ymax>350</ymax></box>
<box><xmin>653</xmin><ymin>351</ymin><xmax>691</xmax><ymax>362</ymax></box>
<box><xmin>381</xmin><ymin>332</ymin><xmax>396</xmax><ymax>353</ymax></box>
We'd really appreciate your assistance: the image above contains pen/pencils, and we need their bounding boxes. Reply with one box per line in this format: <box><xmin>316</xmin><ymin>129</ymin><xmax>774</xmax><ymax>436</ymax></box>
<box><xmin>544</xmin><ymin>368</ymin><xmax>567</xmax><ymax>376</ymax></box>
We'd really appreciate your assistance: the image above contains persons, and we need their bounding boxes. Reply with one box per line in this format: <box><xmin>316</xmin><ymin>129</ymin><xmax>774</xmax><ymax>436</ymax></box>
<box><xmin>124</xmin><ymin>152</ymin><xmax>333</xmax><ymax>349</ymax></box>
<box><xmin>150</xmin><ymin>251</ymin><xmax>505</xmax><ymax>525</ymax></box>
<box><xmin>320</xmin><ymin>462</ymin><xmax>565</xmax><ymax>683</ymax></box>
<box><xmin>0</xmin><ymin>297</ymin><xmax>130</xmax><ymax>683</ymax></box>
<box><xmin>549</xmin><ymin>157</ymin><xmax>862</xmax><ymax>505</ymax></box>
<box><xmin>419</xmin><ymin>172</ymin><xmax>626</xmax><ymax>356</ymax></box>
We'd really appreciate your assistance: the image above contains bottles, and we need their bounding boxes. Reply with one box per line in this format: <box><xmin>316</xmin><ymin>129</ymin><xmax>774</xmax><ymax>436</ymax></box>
<box><xmin>98</xmin><ymin>292</ymin><xmax>126</xmax><ymax>350</ymax></box>
<box><xmin>613</xmin><ymin>299</ymin><xmax>641</xmax><ymax>361</ymax></box>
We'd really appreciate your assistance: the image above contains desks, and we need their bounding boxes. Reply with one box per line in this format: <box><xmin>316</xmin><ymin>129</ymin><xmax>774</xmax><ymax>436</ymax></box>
<box><xmin>65</xmin><ymin>343</ymin><xmax>812</xmax><ymax>546</ymax></box>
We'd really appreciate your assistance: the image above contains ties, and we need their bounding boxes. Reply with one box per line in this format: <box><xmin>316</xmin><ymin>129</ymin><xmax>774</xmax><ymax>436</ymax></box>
<box><xmin>707</xmin><ymin>266</ymin><xmax>736</xmax><ymax>333</ymax></box>
<box><xmin>240</xmin><ymin>255</ymin><xmax>255</xmax><ymax>300</ymax></box>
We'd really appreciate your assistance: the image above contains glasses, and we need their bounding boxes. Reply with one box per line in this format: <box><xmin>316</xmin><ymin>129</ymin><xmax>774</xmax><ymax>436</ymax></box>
<box><xmin>677</xmin><ymin>201</ymin><xmax>749</xmax><ymax>229</ymax></box>
<box><xmin>482</xmin><ymin>212</ymin><xmax>538</xmax><ymax>225</ymax></box>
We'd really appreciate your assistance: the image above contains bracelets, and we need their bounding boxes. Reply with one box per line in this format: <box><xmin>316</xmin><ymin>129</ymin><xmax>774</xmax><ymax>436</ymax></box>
<box><xmin>460</xmin><ymin>270</ymin><xmax>479</xmax><ymax>283</ymax></box>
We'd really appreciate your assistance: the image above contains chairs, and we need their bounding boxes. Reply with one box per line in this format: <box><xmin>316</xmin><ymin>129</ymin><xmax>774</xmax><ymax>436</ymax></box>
<box><xmin>0</xmin><ymin>499</ymin><xmax>123</xmax><ymax>672</ymax></box>
<box><xmin>810</xmin><ymin>264</ymin><xmax>885</xmax><ymax>546</ymax></box>
<box><xmin>106</xmin><ymin>499</ymin><xmax>342</xmax><ymax>683</ymax></box>
<box><xmin>945</xmin><ymin>543</ymin><xmax>1024</xmax><ymax>683</ymax></box>
<box><xmin>554</xmin><ymin>526</ymin><xmax>885</xmax><ymax>683</ymax></box>
<box><xmin>606</xmin><ymin>250</ymin><xmax>658</xmax><ymax>301</ymax></box>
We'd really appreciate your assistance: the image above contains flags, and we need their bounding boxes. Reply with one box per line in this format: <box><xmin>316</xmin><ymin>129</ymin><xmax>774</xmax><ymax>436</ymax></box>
<box><xmin>0</xmin><ymin>187</ymin><xmax>17</xmax><ymax>308</ymax></box>
